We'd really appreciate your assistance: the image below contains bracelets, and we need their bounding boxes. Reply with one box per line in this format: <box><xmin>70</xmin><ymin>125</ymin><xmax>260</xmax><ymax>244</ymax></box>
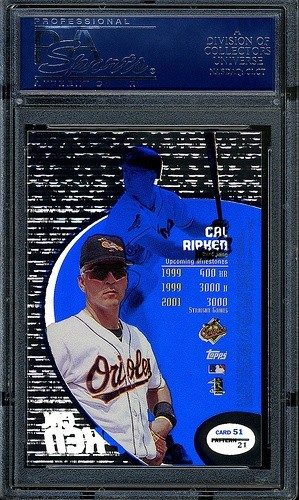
<box><xmin>152</xmin><ymin>401</ymin><xmax>177</xmax><ymax>429</ymax></box>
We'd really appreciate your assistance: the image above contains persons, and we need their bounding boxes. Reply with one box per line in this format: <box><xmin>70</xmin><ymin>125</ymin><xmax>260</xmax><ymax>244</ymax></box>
<box><xmin>49</xmin><ymin>234</ymin><xmax>178</xmax><ymax>468</ymax></box>
<box><xmin>105</xmin><ymin>147</ymin><xmax>232</xmax><ymax>319</ymax></box>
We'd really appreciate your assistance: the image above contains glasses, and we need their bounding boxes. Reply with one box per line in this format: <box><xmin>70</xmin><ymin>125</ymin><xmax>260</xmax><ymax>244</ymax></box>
<box><xmin>81</xmin><ymin>263</ymin><xmax>127</xmax><ymax>282</ymax></box>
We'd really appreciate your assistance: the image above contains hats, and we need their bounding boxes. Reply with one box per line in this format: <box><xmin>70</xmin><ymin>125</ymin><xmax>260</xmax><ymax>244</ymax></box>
<box><xmin>80</xmin><ymin>234</ymin><xmax>135</xmax><ymax>273</ymax></box>
